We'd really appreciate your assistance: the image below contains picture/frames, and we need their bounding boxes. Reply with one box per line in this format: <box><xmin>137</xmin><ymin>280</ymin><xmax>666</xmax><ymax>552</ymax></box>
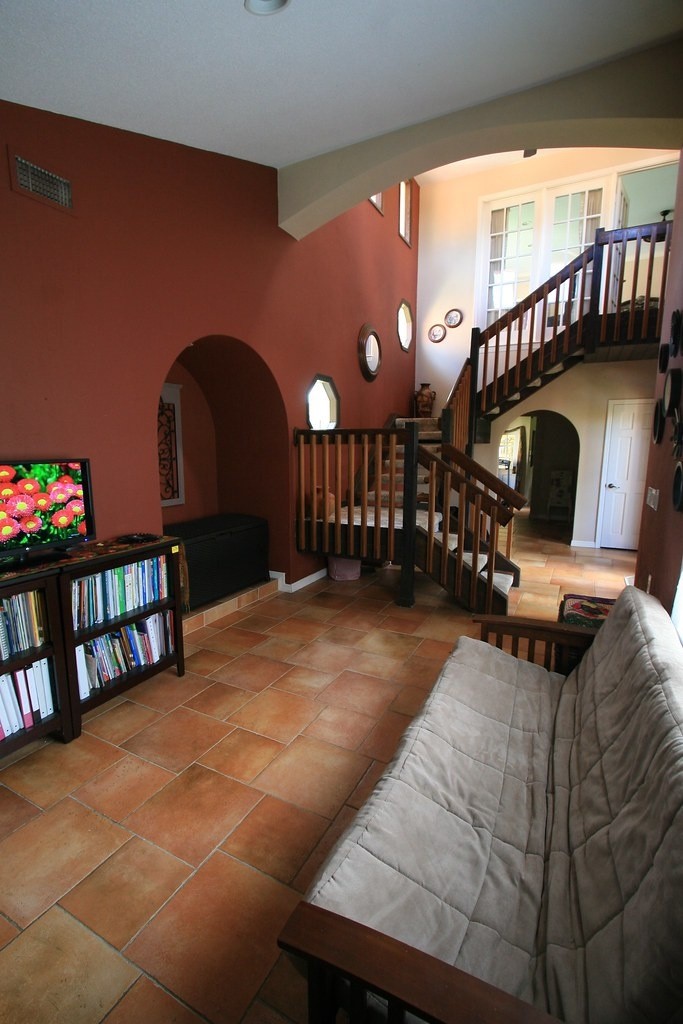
<box><xmin>444</xmin><ymin>309</ymin><xmax>463</xmax><ymax>328</ymax></box>
<box><xmin>652</xmin><ymin>308</ymin><xmax>683</xmax><ymax>512</ymax></box>
<box><xmin>428</xmin><ymin>324</ymin><xmax>446</xmax><ymax>343</ymax></box>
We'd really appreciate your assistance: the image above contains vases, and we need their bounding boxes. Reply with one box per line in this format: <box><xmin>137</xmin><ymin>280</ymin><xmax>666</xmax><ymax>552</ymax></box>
<box><xmin>413</xmin><ymin>383</ymin><xmax>436</xmax><ymax>418</ymax></box>
<box><xmin>311</xmin><ymin>486</ymin><xmax>336</xmax><ymax>518</ymax></box>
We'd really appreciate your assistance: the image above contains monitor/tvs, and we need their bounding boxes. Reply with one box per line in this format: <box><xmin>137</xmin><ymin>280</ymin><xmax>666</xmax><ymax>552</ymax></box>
<box><xmin>0</xmin><ymin>459</ymin><xmax>96</xmax><ymax>573</ymax></box>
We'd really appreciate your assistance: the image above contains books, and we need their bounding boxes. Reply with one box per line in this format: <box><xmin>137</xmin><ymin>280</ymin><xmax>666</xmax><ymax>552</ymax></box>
<box><xmin>0</xmin><ymin>554</ymin><xmax>174</xmax><ymax>741</ymax></box>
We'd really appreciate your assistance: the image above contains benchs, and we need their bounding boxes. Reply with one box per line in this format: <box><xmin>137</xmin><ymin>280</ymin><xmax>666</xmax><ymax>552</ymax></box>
<box><xmin>275</xmin><ymin>587</ymin><xmax>683</xmax><ymax>1024</ymax></box>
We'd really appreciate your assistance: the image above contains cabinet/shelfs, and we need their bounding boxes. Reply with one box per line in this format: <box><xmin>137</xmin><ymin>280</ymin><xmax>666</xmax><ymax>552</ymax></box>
<box><xmin>0</xmin><ymin>532</ymin><xmax>185</xmax><ymax>761</ymax></box>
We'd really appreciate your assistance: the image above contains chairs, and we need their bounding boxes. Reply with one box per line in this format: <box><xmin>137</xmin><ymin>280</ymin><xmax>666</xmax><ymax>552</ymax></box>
<box><xmin>546</xmin><ymin>470</ymin><xmax>573</xmax><ymax>524</ymax></box>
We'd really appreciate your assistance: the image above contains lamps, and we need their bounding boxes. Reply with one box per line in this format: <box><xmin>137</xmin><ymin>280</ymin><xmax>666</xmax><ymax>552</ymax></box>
<box><xmin>643</xmin><ymin>210</ymin><xmax>671</xmax><ymax>243</ymax></box>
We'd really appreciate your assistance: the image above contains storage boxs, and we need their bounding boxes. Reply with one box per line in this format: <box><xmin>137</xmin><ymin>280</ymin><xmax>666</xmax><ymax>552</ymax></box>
<box><xmin>162</xmin><ymin>512</ymin><xmax>269</xmax><ymax>616</ymax></box>
